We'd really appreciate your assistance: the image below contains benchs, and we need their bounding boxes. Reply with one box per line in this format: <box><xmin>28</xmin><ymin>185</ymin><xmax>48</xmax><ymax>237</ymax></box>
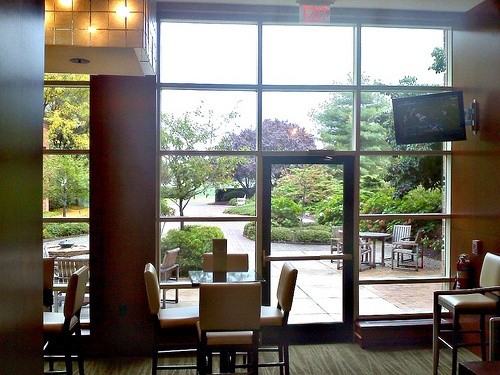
<box><xmin>235</xmin><ymin>194</ymin><xmax>246</xmax><ymax>207</ymax></box>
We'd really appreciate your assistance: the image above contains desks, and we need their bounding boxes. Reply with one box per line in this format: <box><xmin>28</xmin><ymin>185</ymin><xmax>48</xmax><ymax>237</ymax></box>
<box><xmin>359</xmin><ymin>232</ymin><xmax>391</xmax><ymax>268</ymax></box>
<box><xmin>188</xmin><ymin>269</ymin><xmax>266</xmax><ymax>287</ymax></box>
<box><xmin>459</xmin><ymin>361</ymin><xmax>500</xmax><ymax>375</ymax></box>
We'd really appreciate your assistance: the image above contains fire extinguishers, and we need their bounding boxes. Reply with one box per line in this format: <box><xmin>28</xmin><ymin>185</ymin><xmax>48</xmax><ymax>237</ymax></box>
<box><xmin>451</xmin><ymin>253</ymin><xmax>473</xmax><ymax>290</ymax></box>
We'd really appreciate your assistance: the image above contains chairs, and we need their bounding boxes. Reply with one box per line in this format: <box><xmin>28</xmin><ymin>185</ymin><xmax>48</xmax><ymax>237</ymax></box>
<box><xmin>393</xmin><ymin>230</ymin><xmax>425</xmax><ymax>271</ymax></box>
<box><xmin>385</xmin><ymin>225</ymin><xmax>413</xmax><ymax>263</ymax></box>
<box><xmin>161</xmin><ymin>248</ymin><xmax>180</xmax><ymax>309</ymax></box>
<box><xmin>53</xmin><ymin>258</ymin><xmax>90</xmax><ymax>313</ymax></box>
<box><xmin>44</xmin><ymin>265</ymin><xmax>88</xmax><ymax>375</ymax></box>
<box><xmin>197</xmin><ymin>282</ymin><xmax>262</xmax><ymax>374</ymax></box>
<box><xmin>144</xmin><ymin>263</ymin><xmax>199</xmax><ymax>375</ymax></box>
<box><xmin>44</xmin><ymin>257</ymin><xmax>54</xmax><ymax>372</ymax></box>
<box><xmin>331</xmin><ymin>226</ymin><xmax>373</xmax><ymax>271</ymax></box>
<box><xmin>436</xmin><ymin>253</ymin><xmax>500</xmax><ymax>375</ymax></box>
<box><xmin>260</xmin><ymin>261</ymin><xmax>298</xmax><ymax>375</ymax></box>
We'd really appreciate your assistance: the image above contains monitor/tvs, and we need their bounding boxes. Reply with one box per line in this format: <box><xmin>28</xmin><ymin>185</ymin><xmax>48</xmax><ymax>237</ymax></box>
<box><xmin>392</xmin><ymin>91</ymin><xmax>466</xmax><ymax>144</ymax></box>
